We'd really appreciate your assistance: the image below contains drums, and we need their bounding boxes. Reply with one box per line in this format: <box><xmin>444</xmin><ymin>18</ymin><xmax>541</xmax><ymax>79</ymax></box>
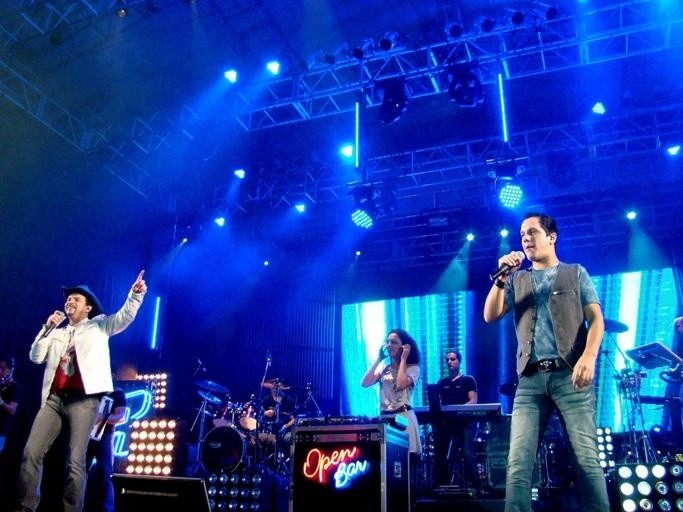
<box><xmin>239</xmin><ymin>400</ymin><xmax>265</xmax><ymax>432</ymax></box>
<box><xmin>201</xmin><ymin>426</ymin><xmax>257</xmax><ymax>475</ymax></box>
<box><xmin>258</xmin><ymin>421</ymin><xmax>277</xmax><ymax>443</ymax></box>
<box><xmin>212</xmin><ymin>402</ymin><xmax>242</xmax><ymax>427</ymax></box>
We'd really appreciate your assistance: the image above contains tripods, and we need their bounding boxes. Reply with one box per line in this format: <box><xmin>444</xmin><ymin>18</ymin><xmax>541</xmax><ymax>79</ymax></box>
<box><xmin>192</xmin><ymin>385</ymin><xmax>289</xmax><ymax>475</ymax></box>
<box><xmin>618</xmin><ymin>390</ymin><xmax>658</xmax><ymax>464</ymax></box>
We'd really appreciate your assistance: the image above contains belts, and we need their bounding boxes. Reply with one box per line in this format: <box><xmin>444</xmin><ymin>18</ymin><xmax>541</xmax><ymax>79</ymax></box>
<box><xmin>380</xmin><ymin>404</ymin><xmax>411</xmax><ymax>414</ymax></box>
<box><xmin>525</xmin><ymin>358</ymin><xmax>566</xmax><ymax>377</ymax></box>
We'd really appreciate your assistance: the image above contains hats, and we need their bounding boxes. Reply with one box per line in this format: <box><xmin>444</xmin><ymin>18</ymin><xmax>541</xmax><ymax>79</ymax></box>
<box><xmin>62</xmin><ymin>285</ymin><xmax>103</xmax><ymax>314</ymax></box>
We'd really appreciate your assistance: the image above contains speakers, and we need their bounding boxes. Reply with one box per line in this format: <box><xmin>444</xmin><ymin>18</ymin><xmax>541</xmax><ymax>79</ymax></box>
<box><xmin>110</xmin><ymin>473</ymin><xmax>212</xmax><ymax>512</ymax></box>
<box><xmin>289</xmin><ymin>423</ymin><xmax>410</xmax><ymax>512</ymax></box>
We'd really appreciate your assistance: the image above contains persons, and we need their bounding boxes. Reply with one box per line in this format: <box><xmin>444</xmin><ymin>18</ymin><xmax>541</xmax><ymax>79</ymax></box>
<box><xmin>484</xmin><ymin>212</ymin><xmax>610</xmax><ymax>511</ymax></box>
<box><xmin>361</xmin><ymin>328</ymin><xmax>423</xmax><ymax>453</ymax></box>
<box><xmin>436</xmin><ymin>350</ymin><xmax>479</xmax><ymax>487</ymax></box>
<box><xmin>18</xmin><ymin>268</ymin><xmax>148</xmax><ymax>511</ymax></box>
<box><xmin>248</xmin><ymin>379</ymin><xmax>296</xmax><ymax>450</ymax></box>
<box><xmin>666</xmin><ymin>316</ymin><xmax>683</xmax><ymax>382</ymax></box>
<box><xmin>0</xmin><ymin>351</ymin><xmax>18</xmax><ymax>460</ymax></box>
<box><xmin>85</xmin><ymin>359</ymin><xmax>126</xmax><ymax>511</ymax></box>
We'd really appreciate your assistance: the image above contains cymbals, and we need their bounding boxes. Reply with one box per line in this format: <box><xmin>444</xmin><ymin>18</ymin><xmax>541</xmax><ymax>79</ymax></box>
<box><xmin>198</xmin><ymin>390</ymin><xmax>222</xmax><ymax>405</ymax></box>
<box><xmin>603</xmin><ymin>318</ymin><xmax>628</xmax><ymax>333</ymax></box>
<box><xmin>260</xmin><ymin>380</ymin><xmax>292</xmax><ymax>389</ymax></box>
<box><xmin>195</xmin><ymin>379</ymin><xmax>229</xmax><ymax>393</ymax></box>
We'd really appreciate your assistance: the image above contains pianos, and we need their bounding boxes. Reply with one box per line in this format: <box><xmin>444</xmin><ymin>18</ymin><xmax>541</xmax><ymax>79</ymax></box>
<box><xmin>413</xmin><ymin>403</ymin><xmax>506</xmax><ymax>425</ymax></box>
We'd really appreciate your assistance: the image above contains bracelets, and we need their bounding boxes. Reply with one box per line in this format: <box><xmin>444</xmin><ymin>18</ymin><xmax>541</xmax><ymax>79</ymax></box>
<box><xmin>495</xmin><ymin>278</ymin><xmax>509</xmax><ymax>289</ymax></box>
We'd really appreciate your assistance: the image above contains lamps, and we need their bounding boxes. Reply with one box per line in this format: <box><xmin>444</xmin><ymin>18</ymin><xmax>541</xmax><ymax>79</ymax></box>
<box><xmin>372</xmin><ymin>59</ymin><xmax>486</xmax><ymax>125</ymax></box>
<box><xmin>346</xmin><ymin>167</ymin><xmax>396</xmax><ymax>230</ymax></box>
<box><xmin>486</xmin><ymin>152</ymin><xmax>531</xmax><ymax>210</ymax></box>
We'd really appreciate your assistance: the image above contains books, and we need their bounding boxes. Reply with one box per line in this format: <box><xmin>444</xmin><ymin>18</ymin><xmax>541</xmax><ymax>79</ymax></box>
<box><xmin>624</xmin><ymin>341</ymin><xmax>682</xmax><ymax>369</ymax></box>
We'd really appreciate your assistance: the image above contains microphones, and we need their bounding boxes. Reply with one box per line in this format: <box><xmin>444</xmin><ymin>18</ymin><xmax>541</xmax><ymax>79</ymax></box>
<box><xmin>43</xmin><ymin>311</ymin><xmax>65</xmax><ymax>338</ymax></box>
<box><xmin>489</xmin><ymin>251</ymin><xmax>525</xmax><ymax>281</ymax></box>
